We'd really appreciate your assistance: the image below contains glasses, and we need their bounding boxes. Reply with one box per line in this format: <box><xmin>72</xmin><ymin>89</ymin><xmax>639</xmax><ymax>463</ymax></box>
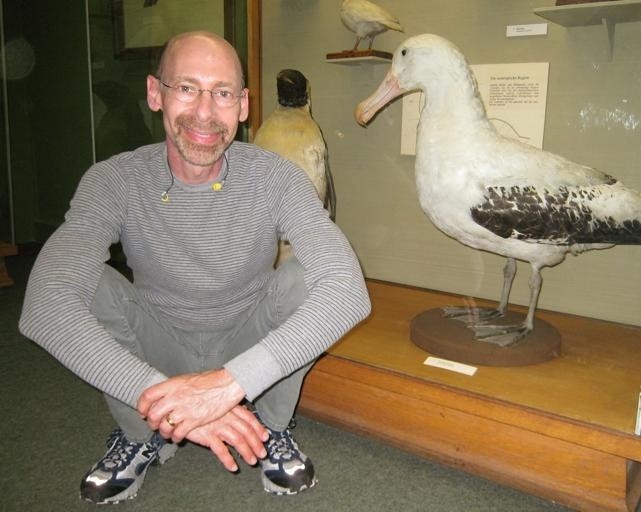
<box><xmin>157</xmin><ymin>77</ymin><xmax>245</xmax><ymax>110</ymax></box>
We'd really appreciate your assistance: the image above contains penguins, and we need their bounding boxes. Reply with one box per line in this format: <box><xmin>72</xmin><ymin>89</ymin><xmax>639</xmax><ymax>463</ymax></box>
<box><xmin>251</xmin><ymin>66</ymin><xmax>338</xmax><ymax>269</ymax></box>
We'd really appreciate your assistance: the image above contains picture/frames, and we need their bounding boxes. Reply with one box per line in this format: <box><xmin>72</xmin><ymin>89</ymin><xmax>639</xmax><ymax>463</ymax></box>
<box><xmin>108</xmin><ymin>0</ymin><xmax>239</xmax><ymax>62</ymax></box>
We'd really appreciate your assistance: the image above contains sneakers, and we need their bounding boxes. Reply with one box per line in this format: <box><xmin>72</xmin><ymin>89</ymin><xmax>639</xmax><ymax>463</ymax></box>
<box><xmin>251</xmin><ymin>413</ymin><xmax>317</xmax><ymax>497</ymax></box>
<box><xmin>78</xmin><ymin>425</ymin><xmax>179</xmax><ymax>507</ymax></box>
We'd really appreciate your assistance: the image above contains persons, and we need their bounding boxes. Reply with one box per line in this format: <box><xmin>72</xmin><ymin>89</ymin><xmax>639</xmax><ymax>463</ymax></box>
<box><xmin>14</xmin><ymin>28</ymin><xmax>376</xmax><ymax>508</ymax></box>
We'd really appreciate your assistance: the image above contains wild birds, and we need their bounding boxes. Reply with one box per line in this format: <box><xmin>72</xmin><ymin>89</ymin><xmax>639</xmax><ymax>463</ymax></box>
<box><xmin>339</xmin><ymin>1</ymin><xmax>405</xmax><ymax>52</ymax></box>
<box><xmin>355</xmin><ymin>32</ymin><xmax>641</xmax><ymax>348</ymax></box>
